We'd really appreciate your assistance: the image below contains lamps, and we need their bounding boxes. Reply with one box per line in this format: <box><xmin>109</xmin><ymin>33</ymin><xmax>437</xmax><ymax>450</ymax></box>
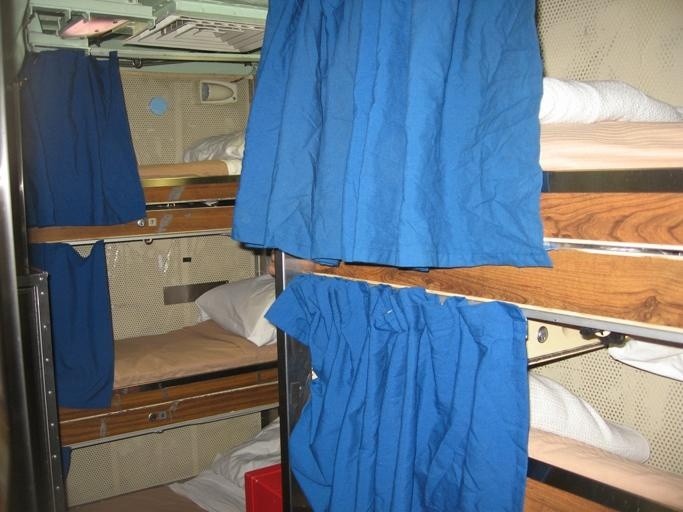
<box><xmin>199</xmin><ymin>80</ymin><xmax>238</xmax><ymax>103</ymax></box>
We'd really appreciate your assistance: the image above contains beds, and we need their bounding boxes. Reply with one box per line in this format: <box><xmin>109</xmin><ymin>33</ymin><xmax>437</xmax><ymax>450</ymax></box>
<box><xmin>274</xmin><ymin>0</ymin><xmax>682</xmax><ymax>512</ymax></box>
<box><xmin>0</xmin><ymin>46</ymin><xmax>285</xmax><ymax>512</ymax></box>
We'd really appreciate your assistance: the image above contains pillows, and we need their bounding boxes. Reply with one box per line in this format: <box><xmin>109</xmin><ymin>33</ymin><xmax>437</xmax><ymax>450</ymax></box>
<box><xmin>183</xmin><ymin>129</ymin><xmax>245</xmax><ymax>164</ymax></box>
<box><xmin>211</xmin><ymin>416</ymin><xmax>282</xmax><ymax>491</ymax></box>
<box><xmin>538</xmin><ymin>77</ymin><xmax>682</xmax><ymax>125</ymax></box>
<box><xmin>529</xmin><ymin>370</ymin><xmax>651</xmax><ymax>465</ymax></box>
